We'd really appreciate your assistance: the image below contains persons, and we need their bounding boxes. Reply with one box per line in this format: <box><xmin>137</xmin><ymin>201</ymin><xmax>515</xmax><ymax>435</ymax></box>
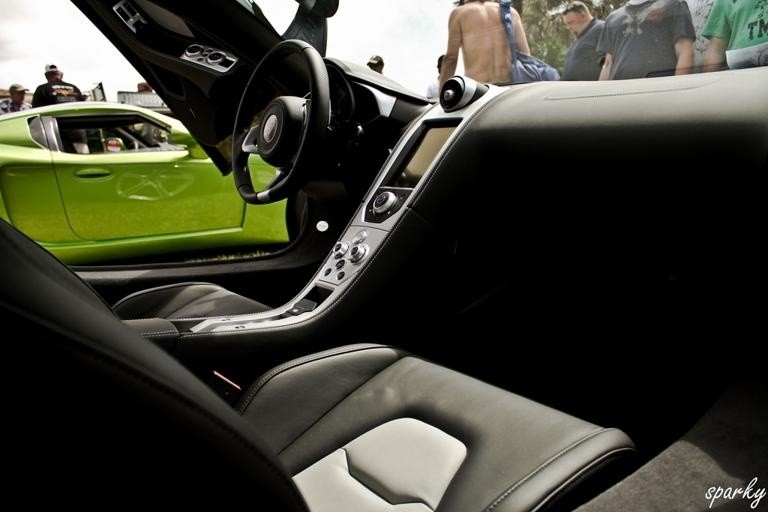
<box><xmin>365</xmin><ymin>56</ymin><xmax>383</xmax><ymax>74</ymax></box>
<box><xmin>557</xmin><ymin>1</ymin><xmax>606</xmax><ymax>81</ymax></box>
<box><xmin>595</xmin><ymin>1</ymin><xmax>697</xmax><ymax>82</ymax></box>
<box><xmin>700</xmin><ymin>1</ymin><xmax>768</xmax><ymax>72</ymax></box>
<box><xmin>29</xmin><ymin>62</ymin><xmax>90</xmax><ymax>154</ymax></box>
<box><xmin>426</xmin><ymin>55</ymin><xmax>445</xmax><ymax>99</ymax></box>
<box><xmin>0</xmin><ymin>83</ymin><xmax>32</xmax><ymax>116</ymax></box>
<box><xmin>437</xmin><ymin>1</ymin><xmax>533</xmax><ymax>89</ymax></box>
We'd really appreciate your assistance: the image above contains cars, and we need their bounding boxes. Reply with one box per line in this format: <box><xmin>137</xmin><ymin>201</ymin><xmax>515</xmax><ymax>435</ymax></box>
<box><xmin>0</xmin><ymin>102</ymin><xmax>290</xmax><ymax>265</ymax></box>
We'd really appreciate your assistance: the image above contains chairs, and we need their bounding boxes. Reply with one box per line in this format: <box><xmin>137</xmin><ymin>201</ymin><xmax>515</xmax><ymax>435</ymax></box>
<box><xmin>0</xmin><ymin>218</ymin><xmax>644</xmax><ymax>512</ymax></box>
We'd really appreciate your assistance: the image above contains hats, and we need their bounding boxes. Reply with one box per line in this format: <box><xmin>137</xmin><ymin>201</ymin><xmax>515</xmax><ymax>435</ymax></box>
<box><xmin>44</xmin><ymin>62</ymin><xmax>63</xmax><ymax>74</ymax></box>
<box><xmin>8</xmin><ymin>83</ymin><xmax>30</xmax><ymax>94</ymax></box>
<box><xmin>365</xmin><ymin>55</ymin><xmax>383</xmax><ymax>67</ymax></box>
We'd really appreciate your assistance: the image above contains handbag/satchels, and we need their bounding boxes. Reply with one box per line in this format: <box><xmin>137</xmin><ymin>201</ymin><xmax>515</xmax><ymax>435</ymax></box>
<box><xmin>508</xmin><ymin>52</ymin><xmax>561</xmax><ymax>85</ymax></box>
